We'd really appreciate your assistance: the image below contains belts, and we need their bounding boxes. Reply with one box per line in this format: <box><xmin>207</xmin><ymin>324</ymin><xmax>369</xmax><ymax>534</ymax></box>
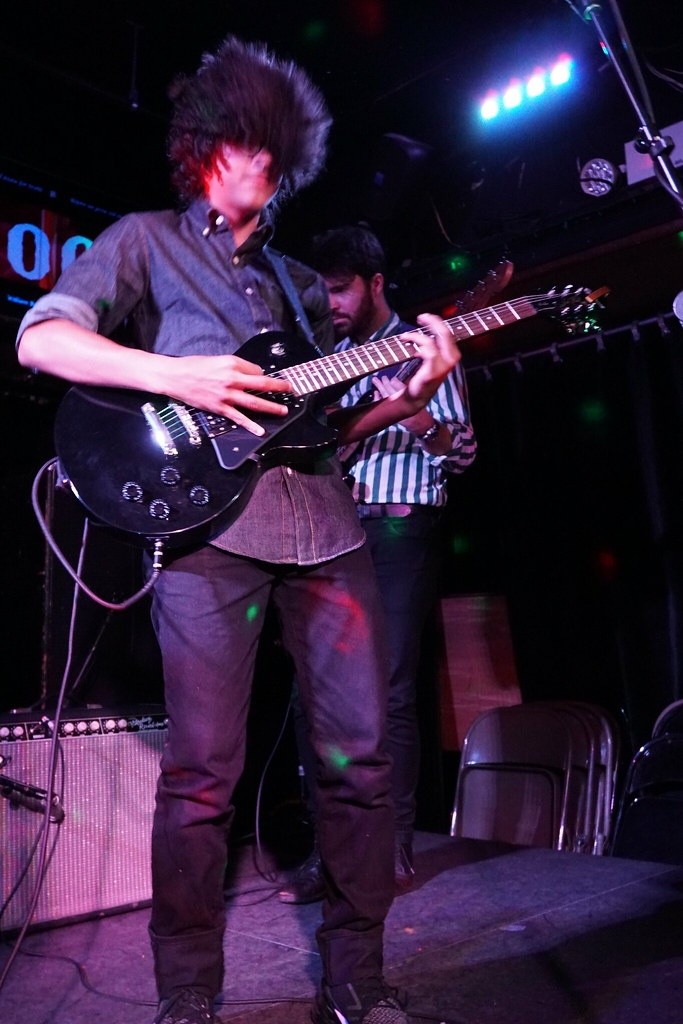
<box><xmin>356</xmin><ymin>503</ymin><xmax>413</xmax><ymax>519</ymax></box>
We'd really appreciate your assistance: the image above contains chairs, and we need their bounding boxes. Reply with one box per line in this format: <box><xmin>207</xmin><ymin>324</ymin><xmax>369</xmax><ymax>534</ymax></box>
<box><xmin>449</xmin><ymin>696</ymin><xmax>683</xmax><ymax>864</ymax></box>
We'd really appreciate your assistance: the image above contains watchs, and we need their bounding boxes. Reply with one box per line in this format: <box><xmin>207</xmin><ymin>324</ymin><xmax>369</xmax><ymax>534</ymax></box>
<box><xmin>419</xmin><ymin>417</ymin><xmax>440</xmax><ymax>446</ymax></box>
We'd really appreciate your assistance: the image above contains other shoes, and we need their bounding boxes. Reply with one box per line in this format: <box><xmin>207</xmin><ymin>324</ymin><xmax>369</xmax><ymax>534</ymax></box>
<box><xmin>310</xmin><ymin>977</ymin><xmax>410</xmax><ymax>1024</ymax></box>
<box><xmin>278</xmin><ymin>841</ymin><xmax>325</xmax><ymax>903</ymax></box>
<box><xmin>395</xmin><ymin>845</ymin><xmax>414</xmax><ymax>885</ymax></box>
<box><xmin>152</xmin><ymin>987</ymin><xmax>214</xmax><ymax>1024</ymax></box>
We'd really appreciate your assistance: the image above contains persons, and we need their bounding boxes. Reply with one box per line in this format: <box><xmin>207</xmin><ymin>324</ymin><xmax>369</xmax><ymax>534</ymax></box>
<box><xmin>269</xmin><ymin>226</ymin><xmax>475</xmax><ymax>905</ymax></box>
<box><xmin>19</xmin><ymin>40</ymin><xmax>463</xmax><ymax>1024</ymax></box>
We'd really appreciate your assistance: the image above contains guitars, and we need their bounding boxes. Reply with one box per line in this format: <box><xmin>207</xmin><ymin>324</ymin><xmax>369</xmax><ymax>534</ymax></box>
<box><xmin>325</xmin><ymin>252</ymin><xmax>519</xmax><ymax>469</ymax></box>
<box><xmin>53</xmin><ymin>276</ymin><xmax>611</xmax><ymax>559</ymax></box>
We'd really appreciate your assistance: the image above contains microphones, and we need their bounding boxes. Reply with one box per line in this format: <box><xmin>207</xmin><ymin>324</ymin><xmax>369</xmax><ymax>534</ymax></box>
<box><xmin>46</xmin><ymin>795</ymin><xmax>65</xmax><ymax>823</ymax></box>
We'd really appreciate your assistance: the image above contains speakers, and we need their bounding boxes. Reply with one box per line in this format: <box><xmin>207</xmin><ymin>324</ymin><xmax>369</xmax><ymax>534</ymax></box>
<box><xmin>0</xmin><ymin>727</ymin><xmax>169</xmax><ymax>932</ymax></box>
